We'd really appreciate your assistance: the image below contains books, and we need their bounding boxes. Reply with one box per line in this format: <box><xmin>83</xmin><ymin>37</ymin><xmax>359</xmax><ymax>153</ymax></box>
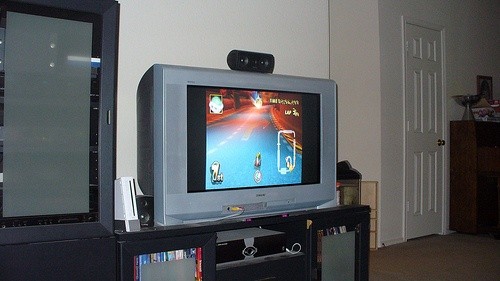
<box><xmin>315</xmin><ymin>225</ymin><xmax>349</xmax><ymax>263</ymax></box>
<box><xmin>132</xmin><ymin>248</ymin><xmax>203</xmax><ymax>281</ymax></box>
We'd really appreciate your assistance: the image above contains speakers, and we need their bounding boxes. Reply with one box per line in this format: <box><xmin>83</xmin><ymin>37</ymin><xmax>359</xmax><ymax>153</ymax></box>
<box><xmin>227</xmin><ymin>50</ymin><xmax>275</xmax><ymax>73</ymax></box>
<box><xmin>136</xmin><ymin>195</ymin><xmax>154</xmax><ymax>228</ymax></box>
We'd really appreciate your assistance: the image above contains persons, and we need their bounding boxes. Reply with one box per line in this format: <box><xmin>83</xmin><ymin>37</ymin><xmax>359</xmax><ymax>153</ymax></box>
<box><xmin>481</xmin><ymin>79</ymin><xmax>490</xmax><ymax>99</ymax></box>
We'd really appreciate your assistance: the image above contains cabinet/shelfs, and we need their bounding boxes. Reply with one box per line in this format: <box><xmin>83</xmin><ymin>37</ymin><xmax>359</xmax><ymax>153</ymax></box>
<box><xmin>450</xmin><ymin>120</ymin><xmax>500</xmax><ymax>233</ymax></box>
<box><xmin>116</xmin><ymin>204</ymin><xmax>371</xmax><ymax>281</ymax></box>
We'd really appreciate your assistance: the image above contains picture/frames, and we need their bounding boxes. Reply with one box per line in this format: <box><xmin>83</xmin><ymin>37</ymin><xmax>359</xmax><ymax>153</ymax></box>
<box><xmin>476</xmin><ymin>75</ymin><xmax>493</xmax><ymax>101</ymax></box>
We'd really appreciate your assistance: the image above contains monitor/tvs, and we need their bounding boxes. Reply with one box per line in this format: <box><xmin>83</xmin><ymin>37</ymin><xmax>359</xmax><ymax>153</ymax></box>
<box><xmin>136</xmin><ymin>64</ymin><xmax>338</xmax><ymax>227</ymax></box>
